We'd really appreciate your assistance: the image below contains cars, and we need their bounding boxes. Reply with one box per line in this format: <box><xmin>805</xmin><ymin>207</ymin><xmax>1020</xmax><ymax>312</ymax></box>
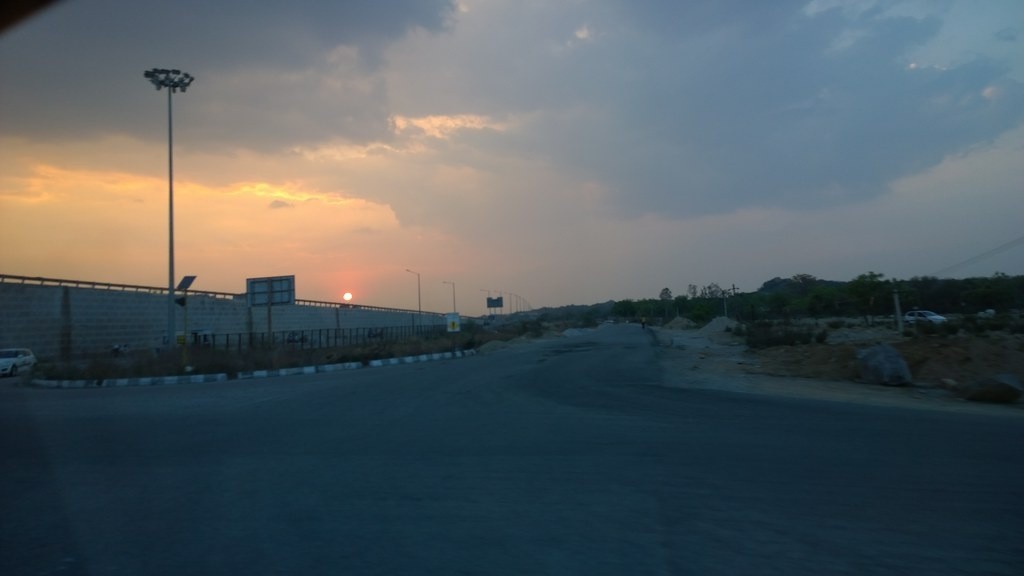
<box><xmin>287</xmin><ymin>331</ymin><xmax>304</xmax><ymax>342</ymax></box>
<box><xmin>367</xmin><ymin>327</ymin><xmax>384</xmax><ymax>337</ymax></box>
<box><xmin>0</xmin><ymin>349</ymin><xmax>37</xmax><ymax>377</ymax></box>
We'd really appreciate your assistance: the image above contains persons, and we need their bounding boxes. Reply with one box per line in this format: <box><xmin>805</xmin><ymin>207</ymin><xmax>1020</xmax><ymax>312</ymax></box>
<box><xmin>640</xmin><ymin>314</ymin><xmax>648</xmax><ymax>330</ymax></box>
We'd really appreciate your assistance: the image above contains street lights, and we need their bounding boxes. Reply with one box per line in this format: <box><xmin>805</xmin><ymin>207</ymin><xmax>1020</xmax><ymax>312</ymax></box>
<box><xmin>406</xmin><ymin>268</ymin><xmax>421</xmax><ymax>324</ymax></box>
<box><xmin>480</xmin><ymin>288</ymin><xmax>532</xmax><ymax>313</ymax></box>
<box><xmin>443</xmin><ymin>281</ymin><xmax>456</xmax><ymax>313</ymax></box>
<box><xmin>143</xmin><ymin>68</ymin><xmax>195</xmax><ymax>342</ymax></box>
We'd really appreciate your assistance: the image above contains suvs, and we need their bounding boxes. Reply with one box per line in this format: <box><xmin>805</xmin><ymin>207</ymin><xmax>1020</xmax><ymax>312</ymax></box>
<box><xmin>904</xmin><ymin>311</ymin><xmax>946</xmax><ymax>325</ymax></box>
